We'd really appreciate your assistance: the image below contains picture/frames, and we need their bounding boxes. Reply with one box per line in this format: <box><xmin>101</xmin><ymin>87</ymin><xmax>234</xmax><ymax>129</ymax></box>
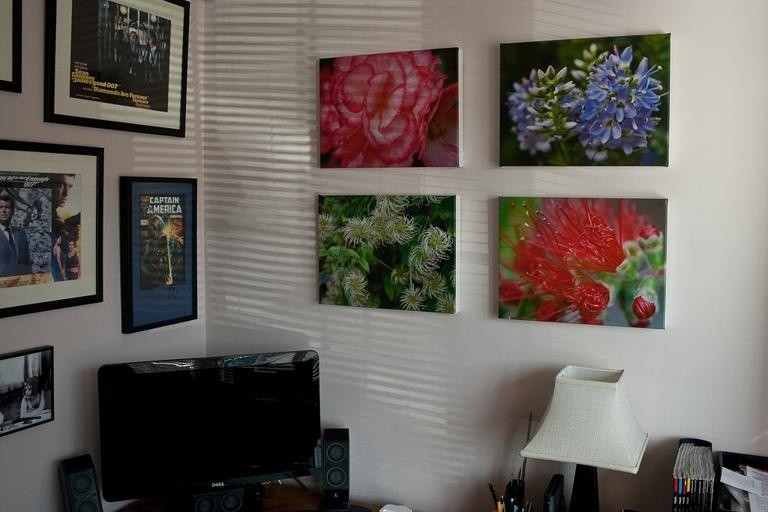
<box><xmin>43</xmin><ymin>1</ymin><xmax>192</xmax><ymax>140</ymax></box>
<box><xmin>0</xmin><ymin>138</ymin><xmax>105</xmax><ymax>318</ymax></box>
<box><xmin>1</xmin><ymin>344</ymin><xmax>55</xmax><ymax>434</ymax></box>
<box><xmin>0</xmin><ymin>0</ymin><xmax>23</xmax><ymax>94</ymax></box>
<box><xmin>118</xmin><ymin>174</ymin><xmax>195</xmax><ymax>334</ymax></box>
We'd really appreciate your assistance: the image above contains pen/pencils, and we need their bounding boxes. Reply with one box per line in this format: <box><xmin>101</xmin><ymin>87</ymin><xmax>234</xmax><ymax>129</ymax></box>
<box><xmin>488</xmin><ymin>479</ymin><xmax>534</xmax><ymax>512</ymax></box>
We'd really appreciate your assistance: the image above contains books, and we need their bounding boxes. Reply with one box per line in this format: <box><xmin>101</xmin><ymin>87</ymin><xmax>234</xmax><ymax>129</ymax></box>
<box><xmin>671</xmin><ymin>442</ymin><xmax>767</xmax><ymax>512</ymax></box>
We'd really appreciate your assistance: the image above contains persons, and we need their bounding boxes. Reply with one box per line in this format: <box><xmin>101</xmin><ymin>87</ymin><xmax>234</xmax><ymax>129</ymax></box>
<box><xmin>146</xmin><ymin>30</ymin><xmax>166</xmax><ymax>85</ymax></box>
<box><xmin>48</xmin><ymin>174</ymin><xmax>75</xmax><ymax>240</ymax></box>
<box><xmin>20</xmin><ymin>376</ymin><xmax>46</xmax><ymax>419</ymax></box>
<box><xmin>0</xmin><ymin>194</ymin><xmax>32</xmax><ymax>278</ymax></box>
<box><xmin>64</xmin><ymin>241</ymin><xmax>78</xmax><ymax>279</ymax></box>
<box><xmin>127</xmin><ymin>31</ymin><xmax>142</xmax><ymax>57</ymax></box>
<box><xmin>50</xmin><ymin>234</ymin><xmax>66</xmax><ymax>281</ymax></box>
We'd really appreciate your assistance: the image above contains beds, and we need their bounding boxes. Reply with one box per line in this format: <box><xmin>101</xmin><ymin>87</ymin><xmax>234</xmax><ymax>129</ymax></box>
<box><xmin>315</xmin><ymin>50</ymin><xmax>458</xmax><ymax>168</ymax></box>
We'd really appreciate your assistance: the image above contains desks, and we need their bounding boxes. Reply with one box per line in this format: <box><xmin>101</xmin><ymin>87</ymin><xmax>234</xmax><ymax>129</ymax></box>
<box><xmin>114</xmin><ymin>479</ymin><xmax>380</xmax><ymax>512</ymax></box>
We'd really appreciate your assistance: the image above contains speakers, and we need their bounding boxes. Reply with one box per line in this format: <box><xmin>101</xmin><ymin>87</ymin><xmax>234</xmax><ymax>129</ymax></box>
<box><xmin>165</xmin><ymin>484</ymin><xmax>264</xmax><ymax>512</ymax></box>
<box><xmin>58</xmin><ymin>454</ymin><xmax>102</xmax><ymax>512</ymax></box>
<box><xmin>322</xmin><ymin>429</ymin><xmax>350</xmax><ymax>512</ymax></box>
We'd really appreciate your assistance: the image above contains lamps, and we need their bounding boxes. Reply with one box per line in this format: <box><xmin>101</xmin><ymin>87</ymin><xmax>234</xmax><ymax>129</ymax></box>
<box><xmin>518</xmin><ymin>364</ymin><xmax>653</xmax><ymax>512</ymax></box>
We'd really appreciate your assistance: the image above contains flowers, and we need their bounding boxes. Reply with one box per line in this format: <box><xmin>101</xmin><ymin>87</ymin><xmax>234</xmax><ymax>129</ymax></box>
<box><xmin>314</xmin><ymin>192</ymin><xmax>455</xmax><ymax>315</ymax></box>
<box><xmin>500</xmin><ymin>39</ymin><xmax>669</xmax><ymax>164</ymax></box>
<box><xmin>496</xmin><ymin>195</ymin><xmax>666</xmax><ymax>333</ymax></box>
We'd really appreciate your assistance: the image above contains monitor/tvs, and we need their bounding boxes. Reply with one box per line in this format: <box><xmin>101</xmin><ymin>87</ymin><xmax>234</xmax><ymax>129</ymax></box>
<box><xmin>97</xmin><ymin>349</ymin><xmax>321</xmax><ymax>502</ymax></box>
